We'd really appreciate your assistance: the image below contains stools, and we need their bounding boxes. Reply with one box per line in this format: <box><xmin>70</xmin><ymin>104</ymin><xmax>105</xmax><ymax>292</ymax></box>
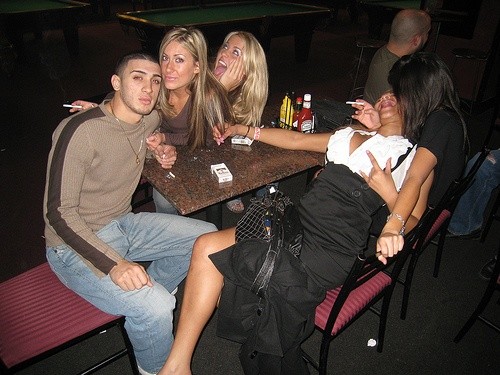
<box><xmin>349</xmin><ymin>37</ymin><xmax>390</xmax><ymax>102</ymax></box>
<box><xmin>449</xmin><ymin>46</ymin><xmax>489</xmax><ymax>103</ymax></box>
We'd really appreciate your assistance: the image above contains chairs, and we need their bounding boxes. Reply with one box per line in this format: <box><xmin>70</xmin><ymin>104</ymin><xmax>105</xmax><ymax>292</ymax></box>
<box><xmin>478</xmin><ymin>192</ymin><xmax>500</xmax><ymax>244</ymax></box>
<box><xmin>0</xmin><ymin>261</ymin><xmax>141</xmax><ymax>375</ymax></box>
<box><xmin>296</xmin><ymin>202</ymin><xmax>437</xmax><ymax>374</ymax></box>
<box><xmin>453</xmin><ymin>246</ymin><xmax>500</xmax><ymax>345</ymax></box>
<box><xmin>481</xmin><ymin>101</ymin><xmax>499</xmax><ymax>150</ymax></box>
<box><xmin>382</xmin><ymin>144</ymin><xmax>492</xmax><ymax>321</ymax></box>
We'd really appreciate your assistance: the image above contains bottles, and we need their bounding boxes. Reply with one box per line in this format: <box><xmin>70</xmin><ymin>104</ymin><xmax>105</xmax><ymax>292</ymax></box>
<box><xmin>292</xmin><ymin>97</ymin><xmax>303</xmax><ymax>131</ymax></box>
<box><xmin>298</xmin><ymin>94</ymin><xmax>316</xmax><ymax>134</ymax></box>
<box><xmin>280</xmin><ymin>92</ymin><xmax>293</xmax><ymax>131</ymax></box>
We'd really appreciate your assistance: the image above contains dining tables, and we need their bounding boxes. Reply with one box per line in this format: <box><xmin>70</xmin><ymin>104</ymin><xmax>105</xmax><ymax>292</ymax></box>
<box><xmin>140</xmin><ymin>85</ymin><xmax>367</xmax><ymax>232</ymax></box>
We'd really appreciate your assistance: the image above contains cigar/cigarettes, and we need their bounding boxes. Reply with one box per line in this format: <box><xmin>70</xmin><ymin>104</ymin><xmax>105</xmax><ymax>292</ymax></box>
<box><xmin>64</xmin><ymin>103</ymin><xmax>83</xmax><ymax>108</ymax></box>
<box><xmin>345</xmin><ymin>101</ymin><xmax>364</xmax><ymax>105</ymax></box>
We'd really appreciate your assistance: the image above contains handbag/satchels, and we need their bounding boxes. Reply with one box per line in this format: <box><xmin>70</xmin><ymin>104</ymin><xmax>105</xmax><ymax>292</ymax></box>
<box><xmin>234</xmin><ymin>184</ymin><xmax>305</xmax><ymax>295</ymax></box>
<box><xmin>313</xmin><ymin>96</ymin><xmax>357</xmax><ymax>131</ymax></box>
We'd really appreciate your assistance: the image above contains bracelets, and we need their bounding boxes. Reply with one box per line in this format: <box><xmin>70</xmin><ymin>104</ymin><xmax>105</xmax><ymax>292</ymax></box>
<box><xmin>386</xmin><ymin>213</ymin><xmax>406</xmax><ymax>234</ymax></box>
<box><xmin>242</xmin><ymin>125</ymin><xmax>251</xmax><ymax>140</ymax></box>
<box><xmin>254</xmin><ymin>126</ymin><xmax>261</xmax><ymax>141</ymax></box>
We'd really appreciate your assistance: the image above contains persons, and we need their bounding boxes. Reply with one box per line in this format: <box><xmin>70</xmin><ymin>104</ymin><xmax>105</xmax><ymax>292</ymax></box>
<box><xmin>209</xmin><ymin>31</ymin><xmax>269</xmax><ymax>129</ymax></box>
<box><xmin>38</xmin><ymin>51</ymin><xmax>220</xmax><ymax>375</ymax></box>
<box><xmin>64</xmin><ymin>25</ymin><xmax>236</xmax><ymax>153</ymax></box>
<box><xmin>430</xmin><ymin>148</ymin><xmax>500</xmax><ymax>287</ymax></box>
<box><xmin>343</xmin><ymin>48</ymin><xmax>473</xmax><ymax>265</ymax></box>
<box><xmin>157</xmin><ymin>87</ymin><xmax>435</xmax><ymax>375</ymax></box>
<box><xmin>363</xmin><ymin>7</ymin><xmax>432</xmax><ymax>106</ymax></box>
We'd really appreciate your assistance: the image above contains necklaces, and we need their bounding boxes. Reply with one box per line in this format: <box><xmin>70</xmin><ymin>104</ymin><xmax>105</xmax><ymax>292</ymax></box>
<box><xmin>108</xmin><ymin>101</ymin><xmax>146</xmax><ymax>170</ymax></box>
